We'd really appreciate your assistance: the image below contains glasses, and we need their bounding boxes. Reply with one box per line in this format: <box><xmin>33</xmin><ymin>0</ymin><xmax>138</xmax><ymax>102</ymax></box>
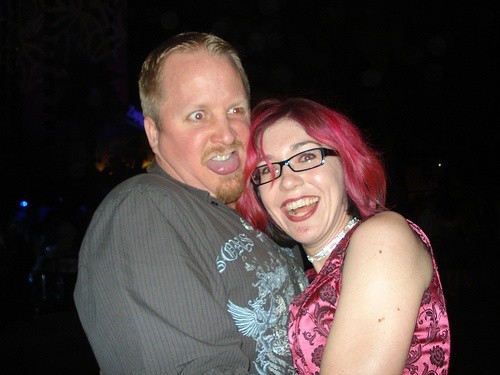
<box><xmin>250</xmin><ymin>147</ymin><xmax>336</xmax><ymax>186</ymax></box>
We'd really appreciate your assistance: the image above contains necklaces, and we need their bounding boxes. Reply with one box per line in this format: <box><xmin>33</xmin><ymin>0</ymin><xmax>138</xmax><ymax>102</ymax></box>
<box><xmin>306</xmin><ymin>216</ymin><xmax>360</xmax><ymax>263</ymax></box>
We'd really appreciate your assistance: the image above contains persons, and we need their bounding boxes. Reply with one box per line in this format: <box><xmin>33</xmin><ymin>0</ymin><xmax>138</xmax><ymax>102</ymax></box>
<box><xmin>74</xmin><ymin>31</ymin><xmax>308</xmax><ymax>375</ymax></box>
<box><xmin>236</xmin><ymin>97</ymin><xmax>450</xmax><ymax>375</ymax></box>
